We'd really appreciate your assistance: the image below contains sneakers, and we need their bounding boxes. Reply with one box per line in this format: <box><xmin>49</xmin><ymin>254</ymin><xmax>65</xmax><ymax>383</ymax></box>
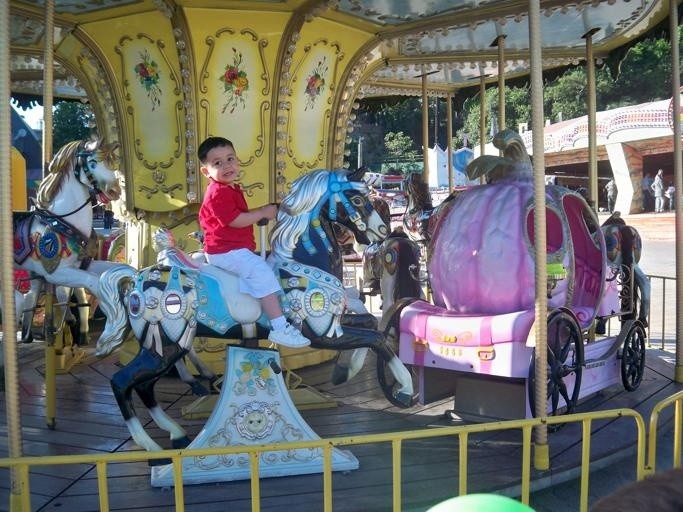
<box><xmin>268</xmin><ymin>322</ymin><xmax>311</xmax><ymax>348</ymax></box>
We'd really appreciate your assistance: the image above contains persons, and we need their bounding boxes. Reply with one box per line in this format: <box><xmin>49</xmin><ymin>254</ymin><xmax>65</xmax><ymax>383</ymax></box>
<box><xmin>650</xmin><ymin>168</ymin><xmax>666</xmax><ymax>211</ymax></box>
<box><xmin>195</xmin><ymin>137</ymin><xmax>313</xmax><ymax>350</ymax></box>
<box><xmin>664</xmin><ymin>177</ymin><xmax>676</xmax><ymax>212</ymax></box>
<box><xmin>605</xmin><ymin>178</ymin><xmax>619</xmax><ymax>215</ymax></box>
<box><xmin>641</xmin><ymin>171</ymin><xmax>652</xmax><ymax>210</ymax></box>
